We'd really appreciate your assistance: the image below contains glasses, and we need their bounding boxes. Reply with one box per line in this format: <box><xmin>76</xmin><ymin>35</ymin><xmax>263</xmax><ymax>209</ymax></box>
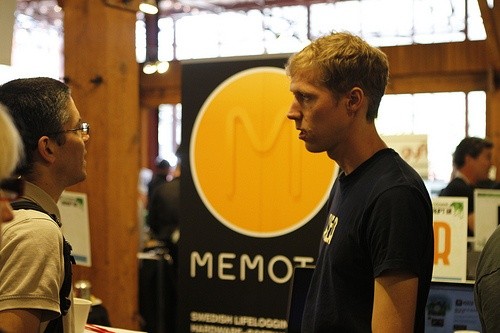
<box><xmin>0</xmin><ymin>177</ymin><xmax>24</xmax><ymax>201</ymax></box>
<box><xmin>33</xmin><ymin>122</ymin><xmax>90</xmax><ymax>146</ymax></box>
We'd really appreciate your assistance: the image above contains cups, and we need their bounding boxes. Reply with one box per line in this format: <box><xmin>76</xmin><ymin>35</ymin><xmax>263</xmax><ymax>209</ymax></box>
<box><xmin>62</xmin><ymin>297</ymin><xmax>92</xmax><ymax>333</ymax></box>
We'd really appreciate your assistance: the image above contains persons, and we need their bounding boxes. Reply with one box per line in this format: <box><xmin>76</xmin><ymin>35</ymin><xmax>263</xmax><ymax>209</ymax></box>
<box><xmin>1</xmin><ymin>104</ymin><xmax>25</xmax><ymax>239</ymax></box>
<box><xmin>284</xmin><ymin>32</ymin><xmax>433</xmax><ymax>333</ymax></box>
<box><xmin>0</xmin><ymin>77</ymin><xmax>91</xmax><ymax>333</ymax></box>
<box><xmin>438</xmin><ymin>136</ymin><xmax>500</xmax><ymax>239</ymax></box>
<box><xmin>147</xmin><ymin>143</ymin><xmax>205</xmax><ymax>332</ymax></box>
